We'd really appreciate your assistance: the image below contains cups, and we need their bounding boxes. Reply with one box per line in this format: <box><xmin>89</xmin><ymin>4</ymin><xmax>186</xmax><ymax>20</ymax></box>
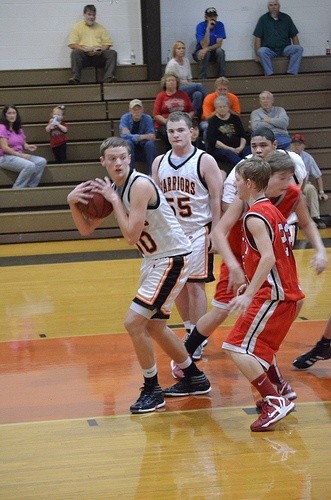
<box><xmin>50</xmin><ymin>117</ymin><xmax>59</xmax><ymax>129</ymax></box>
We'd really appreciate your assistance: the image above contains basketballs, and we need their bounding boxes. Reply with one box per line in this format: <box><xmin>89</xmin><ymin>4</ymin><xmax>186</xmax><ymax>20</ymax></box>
<box><xmin>77</xmin><ymin>180</ymin><xmax>114</xmax><ymax>219</ymax></box>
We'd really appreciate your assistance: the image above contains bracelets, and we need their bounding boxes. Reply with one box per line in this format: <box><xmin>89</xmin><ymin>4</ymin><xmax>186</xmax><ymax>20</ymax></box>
<box><xmin>319</xmin><ymin>190</ymin><xmax>324</xmax><ymax>194</ymax></box>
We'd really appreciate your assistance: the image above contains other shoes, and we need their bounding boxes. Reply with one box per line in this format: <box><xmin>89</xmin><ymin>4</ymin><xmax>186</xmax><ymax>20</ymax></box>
<box><xmin>314</xmin><ymin>218</ymin><xmax>326</xmax><ymax>228</ymax></box>
<box><xmin>104</xmin><ymin>77</ymin><xmax>114</xmax><ymax>83</ymax></box>
<box><xmin>69</xmin><ymin>78</ymin><xmax>79</xmax><ymax>84</ymax></box>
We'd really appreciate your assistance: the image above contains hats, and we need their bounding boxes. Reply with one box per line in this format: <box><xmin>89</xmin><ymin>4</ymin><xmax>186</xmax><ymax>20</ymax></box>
<box><xmin>205</xmin><ymin>7</ymin><xmax>217</xmax><ymax>17</ymax></box>
<box><xmin>290</xmin><ymin>134</ymin><xmax>305</xmax><ymax>143</ymax></box>
<box><xmin>129</xmin><ymin>99</ymin><xmax>143</xmax><ymax>108</ymax></box>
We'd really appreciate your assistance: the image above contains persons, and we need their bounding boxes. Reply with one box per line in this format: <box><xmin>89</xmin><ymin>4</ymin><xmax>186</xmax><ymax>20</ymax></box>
<box><xmin>286</xmin><ymin>133</ymin><xmax>328</xmax><ymax>229</ymax></box>
<box><xmin>293</xmin><ymin>312</ymin><xmax>331</xmax><ymax>369</ymax></box>
<box><xmin>151</xmin><ymin>111</ymin><xmax>223</xmax><ymax>358</ymax></box>
<box><xmin>169</xmin><ymin>151</ymin><xmax>326</xmax><ymax>379</ymax></box>
<box><xmin>253</xmin><ymin>0</ymin><xmax>303</xmax><ymax>76</ymax></box>
<box><xmin>67</xmin><ymin>137</ymin><xmax>213</xmax><ymax>413</ymax></box>
<box><xmin>222</xmin><ymin>155</ymin><xmax>308</xmax><ymax>430</ymax></box>
<box><xmin>151</xmin><ymin>73</ymin><xmax>208</xmax><ymax>154</ymax></box>
<box><xmin>206</xmin><ymin>95</ymin><xmax>248</xmax><ymax>173</ymax></box>
<box><xmin>45</xmin><ymin>105</ymin><xmax>69</xmax><ymax>162</ymax></box>
<box><xmin>192</xmin><ymin>7</ymin><xmax>228</xmax><ymax>79</ymax></box>
<box><xmin>66</xmin><ymin>4</ymin><xmax>118</xmax><ymax>83</ymax></box>
<box><xmin>200</xmin><ymin>77</ymin><xmax>240</xmax><ymax>144</ymax></box>
<box><xmin>221</xmin><ymin>127</ymin><xmax>308</xmax><ymax>247</ymax></box>
<box><xmin>164</xmin><ymin>39</ymin><xmax>208</xmax><ymax>122</ymax></box>
<box><xmin>119</xmin><ymin>99</ymin><xmax>157</xmax><ymax>175</ymax></box>
<box><xmin>249</xmin><ymin>91</ymin><xmax>294</xmax><ymax>150</ymax></box>
<box><xmin>0</xmin><ymin>104</ymin><xmax>47</xmax><ymax>188</ymax></box>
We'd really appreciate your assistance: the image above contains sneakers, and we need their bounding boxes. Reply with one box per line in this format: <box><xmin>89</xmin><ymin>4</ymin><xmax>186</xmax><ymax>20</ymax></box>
<box><xmin>250</xmin><ymin>396</ymin><xmax>295</xmax><ymax>429</ymax></box>
<box><xmin>130</xmin><ymin>382</ymin><xmax>165</xmax><ymax>413</ymax></box>
<box><xmin>163</xmin><ymin>372</ymin><xmax>212</xmax><ymax>397</ymax></box>
<box><xmin>292</xmin><ymin>345</ymin><xmax>331</xmax><ymax>369</ymax></box>
<box><xmin>171</xmin><ymin>360</ymin><xmax>186</xmax><ymax>378</ymax></box>
<box><xmin>256</xmin><ymin>381</ymin><xmax>298</xmax><ymax>406</ymax></box>
<box><xmin>182</xmin><ymin>332</ymin><xmax>208</xmax><ymax>359</ymax></box>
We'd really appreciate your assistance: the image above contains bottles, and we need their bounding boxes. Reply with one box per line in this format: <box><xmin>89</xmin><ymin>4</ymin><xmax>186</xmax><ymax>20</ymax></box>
<box><xmin>167</xmin><ymin>49</ymin><xmax>172</xmax><ymax>63</ymax></box>
<box><xmin>130</xmin><ymin>50</ymin><xmax>136</xmax><ymax>65</ymax></box>
